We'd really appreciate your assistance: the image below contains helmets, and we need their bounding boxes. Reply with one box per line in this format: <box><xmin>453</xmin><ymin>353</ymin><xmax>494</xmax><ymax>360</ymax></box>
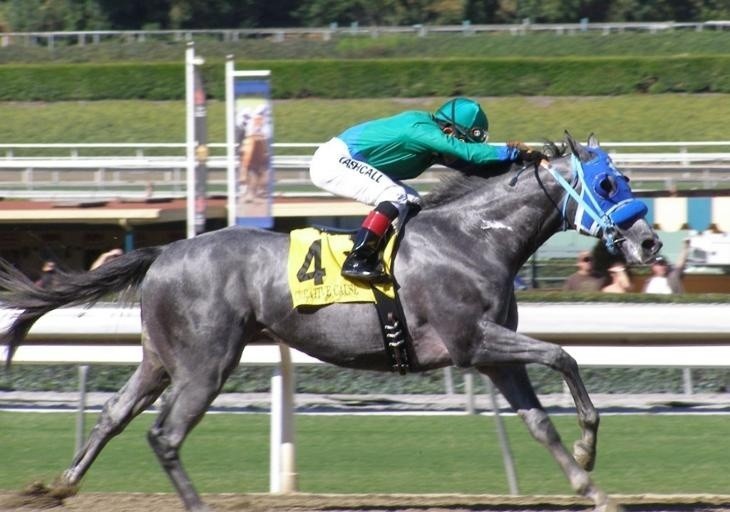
<box><xmin>435</xmin><ymin>98</ymin><xmax>488</xmax><ymax>144</ymax></box>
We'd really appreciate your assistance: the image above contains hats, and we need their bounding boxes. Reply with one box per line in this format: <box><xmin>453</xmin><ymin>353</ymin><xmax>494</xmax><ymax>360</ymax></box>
<box><xmin>652</xmin><ymin>256</ymin><xmax>668</xmax><ymax>267</ymax></box>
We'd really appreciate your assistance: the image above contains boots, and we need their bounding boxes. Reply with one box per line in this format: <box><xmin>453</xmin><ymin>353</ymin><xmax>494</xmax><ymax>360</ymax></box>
<box><xmin>341</xmin><ymin>210</ymin><xmax>395</xmax><ymax>282</ymax></box>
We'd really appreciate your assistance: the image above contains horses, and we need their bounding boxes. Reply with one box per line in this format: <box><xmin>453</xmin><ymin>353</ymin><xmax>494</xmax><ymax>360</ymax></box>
<box><xmin>0</xmin><ymin>127</ymin><xmax>664</xmax><ymax>512</ymax></box>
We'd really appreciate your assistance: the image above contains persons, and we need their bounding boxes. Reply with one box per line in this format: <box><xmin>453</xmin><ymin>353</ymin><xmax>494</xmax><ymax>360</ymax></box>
<box><xmin>563</xmin><ymin>238</ymin><xmax>690</xmax><ymax>294</ymax></box>
<box><xmin>309</xmin><ymin>98</ymin><xmax>533</xmax><ymax>281</ymax></box>
<box><xmin>89</xmin><ymin>248</ymin><xmax>124</xmax><ymax>271</ymax></box>
